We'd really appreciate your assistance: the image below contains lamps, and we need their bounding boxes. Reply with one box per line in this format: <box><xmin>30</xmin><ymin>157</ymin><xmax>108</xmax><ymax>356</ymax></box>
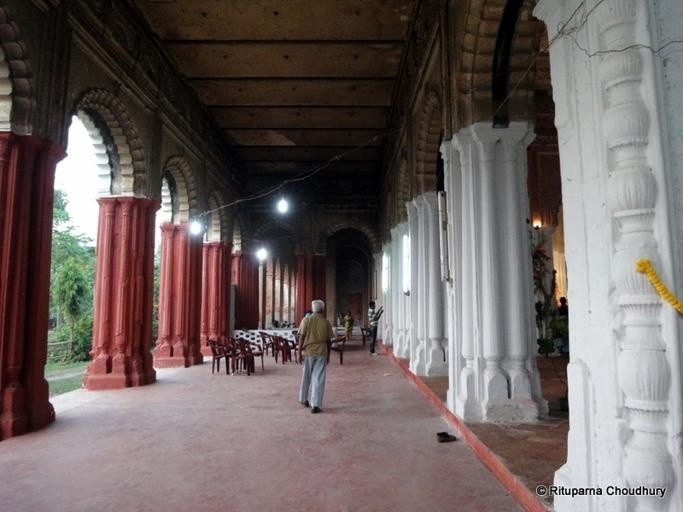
<box><xmin>436</xmin><ymin>190</ymin><xmax>452</xmax><ymax>283</ymax></box>
<box><xmin>401</xmin><ymin>233</ymin><xmax>410</xmax><ymax>296</ymax></box>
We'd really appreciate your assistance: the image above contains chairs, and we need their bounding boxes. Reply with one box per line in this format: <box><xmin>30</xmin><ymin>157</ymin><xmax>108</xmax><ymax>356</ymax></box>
<box><xmin>202</xmin><ymin>324</ymin><xmax>373</xmax><ymax>376</ymax></box>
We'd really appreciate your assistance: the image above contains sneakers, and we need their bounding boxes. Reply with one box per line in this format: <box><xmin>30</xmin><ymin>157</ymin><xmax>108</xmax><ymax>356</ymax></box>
<box><xmin>311</xmin><ymin>406</ymin><xmax>320</xmax><ymax>412</ymax></box>
<box><xmin>437</xmin><ymin>432</ymin><xmax>456</xmax><ymax>443</ymax></box>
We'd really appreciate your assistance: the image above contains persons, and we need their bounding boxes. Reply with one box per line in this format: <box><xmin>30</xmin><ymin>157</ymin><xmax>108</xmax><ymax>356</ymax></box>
<box><xmin>303</xmin><ymin>310</ymin><xmax>313</xmax><ymax>316</ymax></box>
<box><xmin>296</xmin><ymin>299</ymin><xmax>334</xmax><ymax>413</ymax></box>
<box><xmin>367</xmin><ymin>301</ymin><xmax>384</xmax><ymax>356</ymax></box>
<box><xmin>558</xmin><ymin>296</ymin><xmax>567</xmax><ymax>316</ymax></box>
<box><xmin>338</xmin><ymin>310</ymin><xmax>344</xmax><ymax>326</ymax></box>
<box><xmin>343</xmin><ymin>310</ymin><xmax>353</xmax><ymax>341</ymax></box>
<box><xmin>273</xmin><ymin>319</ymin><xmax>297</xmax><ymax>328</ymax></box>
